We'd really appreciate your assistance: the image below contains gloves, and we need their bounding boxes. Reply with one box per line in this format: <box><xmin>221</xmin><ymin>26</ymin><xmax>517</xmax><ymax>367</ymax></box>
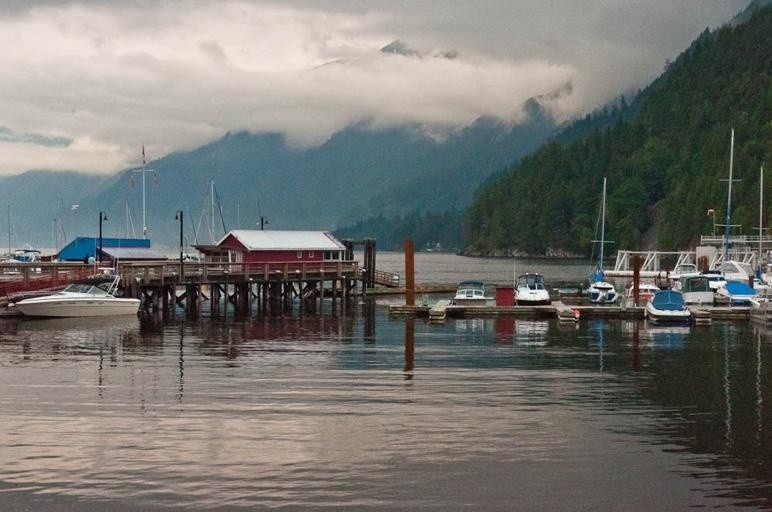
<box><xmin>15</xmin><ymin>272</ymin><xmax>141</xmax><ymax>316</ymax></box>
<box><xmin>646</xmin><ymin>323</ymin><xmax>691</xmax><ymax>351</ymax></box>
<box><xmin>586</xmin><ymin>127</ymin><xmax>772</xmax><ymax>322</ymax></box>
<box><xmin>454</xmin><ymin>280</ymin><xmax>487</xmax><ymax>300</ymax></box>
<box><xmin>621</xmin><ymin>320</ymin><xmax>648</xmax><ymax>350</ymax></box>
<box><xmin>512</xmin><ymin>272</ymin><xmax>551</xmax><ymax>306</ymax></box>
<box><xmin>24</xmin><ymin>314</ymin><xmax>142</xmax><ymax>344</ymax></box>
<box><xmin>514</xmin><ymin>320</ymin><xmax>550</xmax><ymax>348</ymax></box>
<box><xmin>455</xmin><ymin>319</ymin><xmax>486</xmax><ymax>333</ymax></box>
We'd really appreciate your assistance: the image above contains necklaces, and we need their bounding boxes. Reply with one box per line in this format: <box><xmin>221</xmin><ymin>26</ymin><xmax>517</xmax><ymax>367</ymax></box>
<box><xmin>419</xmin><ymin>241</ymin><xmax>433</xmax><ymax>252</ymax></box>
<box><xmin>435</xmin><ymin>241</ymin><xmax>442</xmax><ymax>252</ymax></box>
<box><xmin>0</xmin><ymin>145</ymin><xmax>283</xmax><ymax>277</ymax></box>
<box><xmin>588</xmin><ymin>316</ymin><xmax>611</xmax><ymax>409</ymax></box>
